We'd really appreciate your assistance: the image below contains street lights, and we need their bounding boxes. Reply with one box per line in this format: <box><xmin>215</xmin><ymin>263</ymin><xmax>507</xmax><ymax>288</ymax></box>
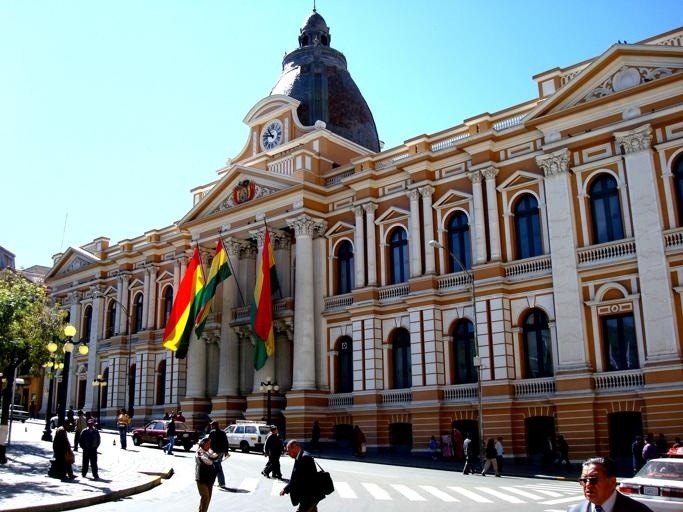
<box><xmin>429</xmin><ymin>240</ymin><xmax>485</xmax><ymax>474</ymax></box>
<box><xmin>93</xmin><ymin>290</ymin><xmax>132</xmax><ymax>416</ymax></box>
<box><xmin>92</xmin><ymin>374</ymin><xmax>108</xmax><ymax>429</ymax></box>
<box><xmin>40</xmin><ymin>355</ymin><xmax>65</xmax><ymax>444</ymax></box>
<box><xmin>259</xmin><ymin>376</ymin><xmax>279</xmax><ymax>424</ymax></box>
<box><xmin>46</xmin><ymin>326</ymin><xmax>89</xmax><ymax>426</ymax></box>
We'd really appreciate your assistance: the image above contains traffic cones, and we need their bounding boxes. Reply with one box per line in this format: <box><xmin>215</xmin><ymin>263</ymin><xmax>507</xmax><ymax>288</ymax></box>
<box><xmin>23</xmin><ymin>426</ymin><xmax>27</xmax><ymax>432</ymax></box>
<box><xmin>112</xmin><ymin>438</ymin><xmax>116</xmax><ymax>445</ymax></box>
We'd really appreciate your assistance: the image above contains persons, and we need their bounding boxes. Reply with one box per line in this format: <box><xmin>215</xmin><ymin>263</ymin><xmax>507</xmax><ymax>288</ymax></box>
<box><xmin>194</xmin><ymin>437</ymin><xmax>220</xmax><ymax>512</ymax></box>
<box><xmin>280</xmin><ymin>440</ymin><xmax>325</xmax><ymax>512</ymax></box>
<box><xmin>428</xmin><ymin>427</ymin><xmax>681</xmax><ymax>478</ymax></box>
<box><xmin>311</xmin><ymin>420</ymin><xmax>321</xmax><ymax>453</ymax></box>
<box><xmin>49</xmin><ymin>403</ymin><xmax>285</xmax><ymax>488</ymax></box>
<box><xmin>569</xmin><ymin>457</ymin><xmax>654</xmax><ymax>511</ymax></box>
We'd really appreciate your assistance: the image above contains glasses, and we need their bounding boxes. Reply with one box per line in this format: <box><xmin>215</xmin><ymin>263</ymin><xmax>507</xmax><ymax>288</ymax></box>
<box><xmin>577</xmin><ymin>475</ymin><xmax>608</xmax><ymax>485</ymax></box>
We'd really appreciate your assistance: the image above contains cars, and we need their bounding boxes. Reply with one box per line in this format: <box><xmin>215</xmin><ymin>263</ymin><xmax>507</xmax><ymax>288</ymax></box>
<box><xmin>616</xmin><ymin>457</ymin><xmax>683</xmax><ymax>512</ymax></box>
<box><xmin>131</xmin><ymin>419</ymin><xmax>198</xmax><ymax>452</ymax></box>
<box><xmin>0</xmin><ymin>403</ymin><xmax>31</xmax><ymax>423</ymax></box>
<box><xmin>50</xmin><ymin>409</ymin><xmax>100</xmax><ymax>430</ymax></box>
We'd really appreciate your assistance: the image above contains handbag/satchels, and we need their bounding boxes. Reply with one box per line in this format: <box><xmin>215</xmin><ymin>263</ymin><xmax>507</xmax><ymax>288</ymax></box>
<box><xmin>316</xmin><ymin>470</ymin><xmax>335</xmax><ymax>495</ymax></box>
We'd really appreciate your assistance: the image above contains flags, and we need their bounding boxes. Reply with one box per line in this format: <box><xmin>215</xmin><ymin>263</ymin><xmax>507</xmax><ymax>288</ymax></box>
<box><xmin>161</xmin><ymin>244</ymin><xmax>206</xmax><ymax>359</ymax></box>
<box><xmin>192</xmin><ymin>238</ymin><xmax>231</xmax><ymax>340</ymax></box>
<box><xmin>248</xmin><ymin>228</ymin><xmax>282</xmax><ymax>371</ymax></box>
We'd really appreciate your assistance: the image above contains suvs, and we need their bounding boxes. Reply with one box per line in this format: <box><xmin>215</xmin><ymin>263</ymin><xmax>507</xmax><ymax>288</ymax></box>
<box><xmin>204</xmin><ymin>418</ymin><xmax>279</xmax><ymax>452</ymax></box>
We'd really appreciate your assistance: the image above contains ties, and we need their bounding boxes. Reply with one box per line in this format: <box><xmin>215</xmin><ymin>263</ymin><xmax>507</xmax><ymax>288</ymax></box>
<box><xmin>592</xmin><ymin>505</ymin><xmax>605</xmax><ymax>512</ymax></box>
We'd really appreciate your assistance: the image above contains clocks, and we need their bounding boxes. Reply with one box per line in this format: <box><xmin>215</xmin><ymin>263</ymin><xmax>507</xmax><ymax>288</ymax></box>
<box><xmin>260</xmin><ymin>119</ymin><xmax>284</xmax><ymax>151</ymax></box>
